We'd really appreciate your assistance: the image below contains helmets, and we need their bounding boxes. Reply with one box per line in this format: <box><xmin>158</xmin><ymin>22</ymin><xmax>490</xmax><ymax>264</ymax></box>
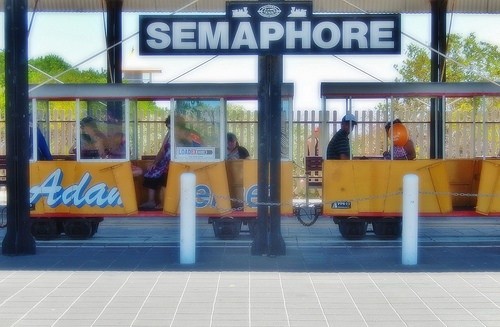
<box><xmin>388</xmin><ymin>124</ymin><xmax>408</xmax><ymax>146</ymax></box>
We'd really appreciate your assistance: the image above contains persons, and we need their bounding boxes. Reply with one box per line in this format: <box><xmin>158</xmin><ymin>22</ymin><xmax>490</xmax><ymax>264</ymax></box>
<box><xmin>29</xmin><ymin>114</ymin><xmax>204</xmax><ymax>211</ymax></box>
<box><xmin>226</xmin><ymin>132</ymin><xmax>250</xmax><ymax>160</ymax></box>
<box><xmin>382</xmin><ymin>119</ymin><xmax>417</xmax><ymax>160</ymax></box>
<box><xmin>307</xmin><ymin>126</ymin><xmax>321</xmax><ymax>181</ymax></box>
<box><xmin>327</xmin><ymin>114</ymin><xmax>358</xmax><ymax>160</ymax></box>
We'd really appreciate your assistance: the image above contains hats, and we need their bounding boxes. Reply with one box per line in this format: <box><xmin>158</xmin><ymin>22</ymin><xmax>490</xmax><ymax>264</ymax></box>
<box><xmin>342</xmin><ymin>114</ymin><xmax>357</xmax><ymax>125</ymax></box>
<box><xmin>80</xmin><ymin>117</ymin><xmax>96</xmax><ymax>124</ymax></box>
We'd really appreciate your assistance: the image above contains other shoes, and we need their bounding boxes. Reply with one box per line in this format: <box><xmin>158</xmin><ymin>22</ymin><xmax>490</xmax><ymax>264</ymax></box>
<box><xmin>139</xmin><ymin>201</ymin><xmax>156</xmax><ymax>209</ymax></box>
<box><xmin>153</xmin><ymin>203</ymin><xmax>164</xmax><ymax>210</ymax></box>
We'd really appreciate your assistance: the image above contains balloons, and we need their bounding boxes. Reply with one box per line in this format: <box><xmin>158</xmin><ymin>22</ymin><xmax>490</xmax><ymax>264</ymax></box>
<box><xmin>388</xmin><ymin>123</ymin><xmax>408</xmax><ymax>148</ymax></box>
